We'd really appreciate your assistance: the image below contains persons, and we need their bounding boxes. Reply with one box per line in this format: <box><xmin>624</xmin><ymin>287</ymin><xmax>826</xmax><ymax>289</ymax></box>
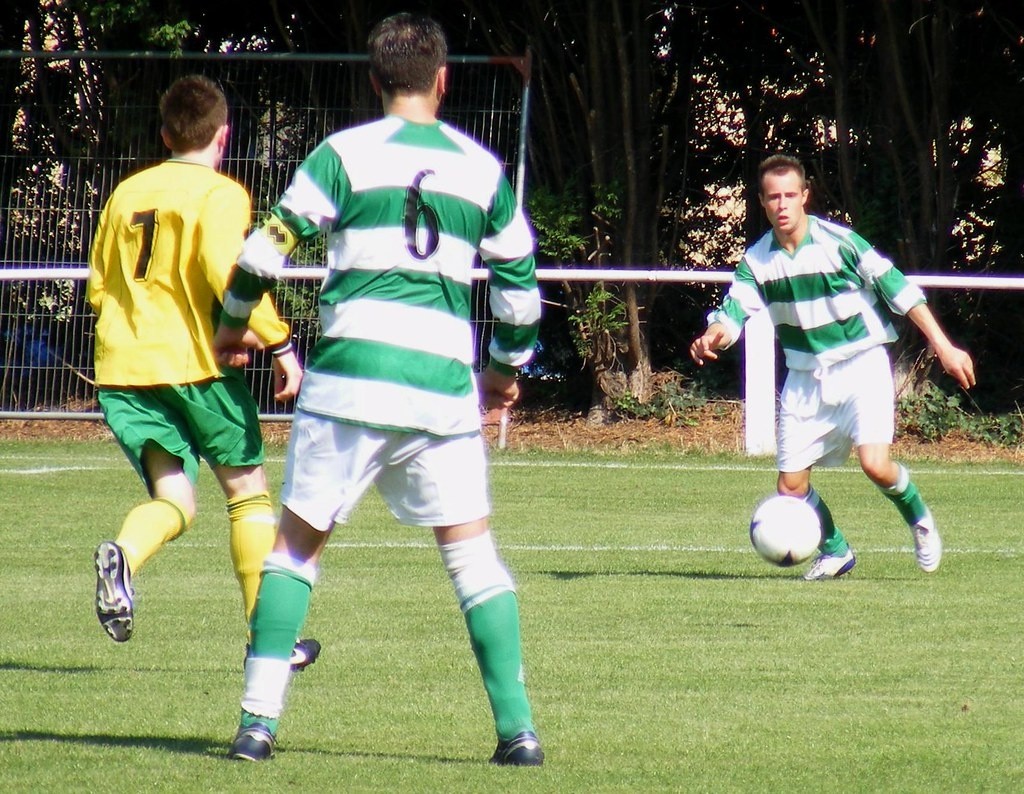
<box><xmin>86</xmin><ymin>75</ymin><xmax>320</xmax><ymax>672</ymax></box>
<box><xmin>214</xmin><ymin>15</ymin><xmax>545</xmax><ymax>767</ymax></box>
<box><xmin>693</xmin><ymin>154</ymin><xmax>976</xmax><ymax>581</ymax></box>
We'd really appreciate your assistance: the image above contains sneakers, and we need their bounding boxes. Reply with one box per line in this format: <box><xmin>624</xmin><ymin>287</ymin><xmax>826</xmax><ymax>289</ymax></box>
<box><xmin>489</xmin><ymin>730</ymin><xmax>546</xmax><ymax>766</ymax></box>
<box><xmin>910</xmin><ymin>505</ymin><xmax>941</xmax><ymax>573</ymax></box>
<box><xmin>93</xmin><ymin>541</ymin><xmax>136</xmax><ymax>643</ymax></box>
<box><xmin>804</xmin><ymin>543</ymin><xmax>856</xmax><ymax>581</ymax></box>
<box><xmin>243</xmin><ymin>635</ymin><xmax>321</xmax><ymax>680</ymax></box>
<box><xmin>230</xmin><ymin>723</ymin><xmax>276</xmax><ymax>763</ymax></box>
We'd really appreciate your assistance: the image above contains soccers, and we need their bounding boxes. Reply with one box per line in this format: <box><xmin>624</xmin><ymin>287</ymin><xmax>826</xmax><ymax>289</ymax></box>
<box><xmin>747</xmin><ymin>496</ymin><xmax>825</xmax><ymax>567</ymax></box>
